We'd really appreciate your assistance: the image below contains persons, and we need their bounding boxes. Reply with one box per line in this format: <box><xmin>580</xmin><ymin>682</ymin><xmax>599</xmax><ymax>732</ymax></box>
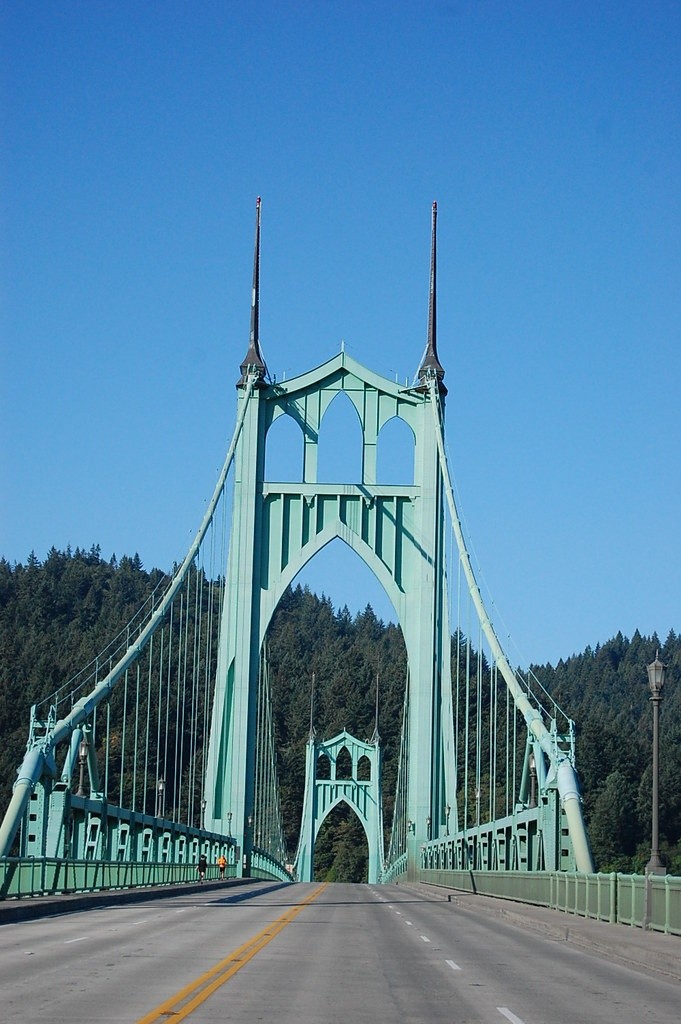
<box><xmin>217</xmin><ymin>855</ymin><xmax>226</xmax><ymax>881</ymax></box>
<box><xmin>194</xmin><ymin>855</ymin><xmax>207</xmax><ymax>884</ymax></box>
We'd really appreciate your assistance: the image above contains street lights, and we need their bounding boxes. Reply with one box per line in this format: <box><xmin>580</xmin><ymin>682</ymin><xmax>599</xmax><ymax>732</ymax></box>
<box><xmin>247</xmin><ymin>816</ymin><xmax>254</xmax><ymax>827</ymax></box>
<box><xmin>443</xmin><ymin>802</ymin><xmax>451</xmax><ymax>837</ymax></box>
<box><xmin>76</xmin><ymin>737</ymin><xmax>89</xmax><ymax>797</ymax></box>
<box><xmin>426</xmin><ymin>816</ymin><xmax>431</xmax><ymax>842</ymax></box>
<box><xmin>226</xmin><ymin>810</ymin><xmax>233</xmax><ymax>838</ymax></box>
<box><xmin>406</xmin><ymin>818</ymin><xmax>415</xmax><ymax>834</ymax></box>
<box><xmin>199</xmin><ymin>798</ymin><xmax>207</xmax><ymax>831</ymax></box>
<box><xmin>528</xmin><ymin>753</ymin><xmax>538</xmax><ymax>808</ymax></box>
<box><xmin>474</xmin><ymin>787</ymin><xmax>480</xmax><ymax>827</ymax></box>
<box><xmin>156</xmin><ymin>776</ymin><xmax>164</xmax><ymax>819</ymax></box>
